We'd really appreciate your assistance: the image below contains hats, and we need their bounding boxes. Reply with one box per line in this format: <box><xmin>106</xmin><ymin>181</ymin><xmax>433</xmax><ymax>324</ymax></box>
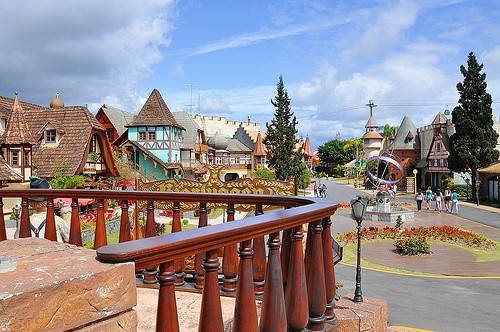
<box><xmin>27</xmin><ymin>176</ymin><xmax>53</xmax><ymax>201</ymax></box>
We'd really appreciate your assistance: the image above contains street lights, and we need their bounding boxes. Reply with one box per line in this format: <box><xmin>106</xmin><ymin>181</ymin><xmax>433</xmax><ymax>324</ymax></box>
<box><xmin>465</xmin><ymin>178</ymin><xmax>470</xmax><ymax>201</ymax></box>
<box><xmin>350</xmin><ymin>197</ymin><xmax>367</xmax><ymax>303</ymax></box>
<box><xmin>476</xmin><ymin>180</ymin><xmax>481</xmax><ymax>207</ymax></box>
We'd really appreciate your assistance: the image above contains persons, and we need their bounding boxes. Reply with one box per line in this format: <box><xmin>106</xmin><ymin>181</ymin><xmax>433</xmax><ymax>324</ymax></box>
<box><xmin>451</xmin><ymin>190</ymin><xmax>461</xmax><ymax>215</ymax></box>
<box><xmin>433</xmin><ymin>189</ymin><xmax>444</xmax><ymax>212</ymax></box>
<box><xmin>444</xmin><ymin>186</ymin><xmax>452</xmax><ymax>214</ymax></box>
<box><xmin>414</xmin><ymin>189</ymin><xmax>423</xmax><ymax>212</ymax></box>
<box><xmin>392</xmin><ymin>184</ymin><xmax>397</xmax><ymax>198</ymax></box>
<box><xmin>424</xmin><ymin>186</ymin><xmax>433</xmax><ymax>211</ymax></box>
<box><xmin>314</xmin><ymin>183</ymin><xmax>327</xmax><ymax>198</ymax></box>
<box><xmin>14</xmin><ymin>176</ymin><xmax>70</xmax><ymax>243</ymax></box>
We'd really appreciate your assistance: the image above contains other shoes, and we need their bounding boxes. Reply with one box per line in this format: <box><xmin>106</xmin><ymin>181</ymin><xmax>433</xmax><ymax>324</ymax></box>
<box><xmin>418</xmin><ymin>207</ymin><xmax>451</xmax><ymax>213</ymax></box>
